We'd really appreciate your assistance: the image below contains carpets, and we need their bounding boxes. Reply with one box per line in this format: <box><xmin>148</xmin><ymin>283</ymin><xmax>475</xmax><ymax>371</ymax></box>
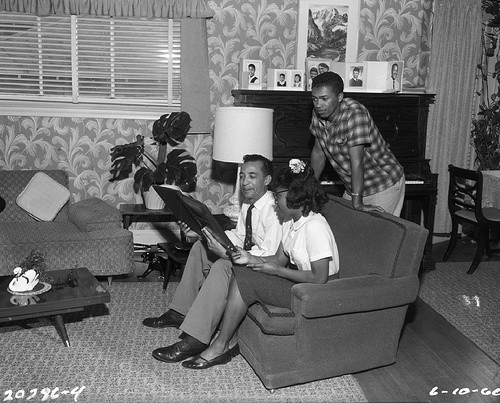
<box><xmin>0</xmin><ymin>280</ymin><xmax>370</xmax><ymax>403</ymax></box>
<box><xmin>419</xmin><ymin>260</ymin><xmax>500</xmax><ymax>367</ymax></box>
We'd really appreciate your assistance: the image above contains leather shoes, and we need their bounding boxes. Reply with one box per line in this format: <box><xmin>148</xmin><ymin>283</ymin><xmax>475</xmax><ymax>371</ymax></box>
<box><xmin>182</xmin><ymin>349</ymin><xmax>231</xmax><ymax>369</ymax></box>
<box><xmin>229</xmin><ymin>343</ymin><xmax>239</xmax><ymax>357</ymax></box>
<box><xmin>152</xmin><ymin>340</ymin><xmax>208</xmax><ymax>363</ymax></box>
<box><xmin>143</xmin><ymin>309</ymin><xmax>185</xmax><ymax>329</ymax></box>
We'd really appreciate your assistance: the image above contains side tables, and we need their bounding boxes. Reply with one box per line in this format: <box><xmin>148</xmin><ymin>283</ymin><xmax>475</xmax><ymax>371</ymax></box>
<box><xmin>158</xmin><ymin>242</ymin><xmax>196</xmax><ymax>294</ymax></box>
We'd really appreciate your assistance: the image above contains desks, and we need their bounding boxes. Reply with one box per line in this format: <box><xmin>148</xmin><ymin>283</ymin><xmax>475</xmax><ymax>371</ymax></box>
<box><xmin>119</xmin><ymin>203</ymin><xmax>189</xmax><ymax>250</ymax></box>
<box><xmin>465</xmin><ymin>169</ymin><xmax>500</xmax><ymax>210</ymax></box>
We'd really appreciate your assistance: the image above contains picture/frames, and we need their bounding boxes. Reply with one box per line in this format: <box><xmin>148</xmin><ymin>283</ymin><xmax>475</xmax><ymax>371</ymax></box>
<box><xmin>296</xmin><ymin>1</ymin><xmax>360</xmax><ymax>73</ymax></box>
<box><xmin>304</xmin><ymin>57</ymin><xmax>332</xmax><ymax>91</ymax></box>
<box><xmin>240</xmin><ymin>57</ymin><xmax>262</xmax><ymax>90</ymax></box>
<box><xmin>274</xmin><ymin>69</ymin><xmax>305</xmax><ymax>91</ymax></box>
<box><xmin>384</xmin><ymin>60</ymin><xmax>404</xmax><ymax>92</ymax></box>
<box><xmin>343</xmin><ymin>62</ymin><xmax>368</xmax><ymax>92</ymax></box>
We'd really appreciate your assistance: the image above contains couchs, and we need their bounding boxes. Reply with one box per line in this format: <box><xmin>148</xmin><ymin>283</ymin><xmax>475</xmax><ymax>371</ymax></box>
<box><xmin>0</xmin><ymin>170</ymin><xmax>135</xmax><ymax>286</ymax></box>
<box><xmin>240</xmin><ymin>193</ymin><xmax>429</xmax><ymax>391</ymax></box>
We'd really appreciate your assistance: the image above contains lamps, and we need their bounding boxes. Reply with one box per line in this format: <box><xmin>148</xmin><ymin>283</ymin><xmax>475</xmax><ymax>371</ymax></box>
<box><xmin>212</xmin><ymin>106</ymin><xmax>275</xmax><ymax>222</ymax></box>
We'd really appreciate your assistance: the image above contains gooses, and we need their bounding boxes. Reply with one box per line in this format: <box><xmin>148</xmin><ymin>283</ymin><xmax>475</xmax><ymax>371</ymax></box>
<box><xmin>8</xmin><ymin>266</ymin><xmax>40</xmax><ymax>292</ymax></box>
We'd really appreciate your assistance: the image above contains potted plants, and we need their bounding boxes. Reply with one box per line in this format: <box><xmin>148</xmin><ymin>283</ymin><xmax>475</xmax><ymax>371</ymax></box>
<box><xmin>109</xmin><ymin>111</ymin><xmax>197</xmax><ymax>210</ymax></box>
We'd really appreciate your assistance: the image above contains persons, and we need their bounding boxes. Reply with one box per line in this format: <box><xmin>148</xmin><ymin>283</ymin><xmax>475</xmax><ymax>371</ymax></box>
<box><xmin>294</xmin><ymin>73</ymin><xmax>302</xmax><ymax>88</ymax></box>
<box><xmin>248</xmin><ymin>64</ymin><xmax>260</xmax><ymax>84</ymax></box>
<box><xmin>349</xmin><ymin>69</ymin><xmax>363</xmax><ymax>87</ymax></box>
<box><xmin>179</xmin><ymin>159</ymin><xmax>342</xmax><ymax>368</ymax></box>
<box><xmin>142</xmin><ymin>154</ymin><xmax>285</xmax><ymax>362</ymax></box>
<box><xmin>277</xmin><ymin>72</ymin><xmax>286</xmax><ymax>86</ymax></box>
<box><xmin>388</xmin><ymin>63</ymin><xmax>401</xmax><ymax>91</ymax></box>
<box><xmin>318</xmin><ymin>63</ymin><xmax>330</xmax><ymax>74</ymax></box>
<box><xmin>309</xmin><ymin>66</ymin><xmax>319</xmax><ymax>89</ymax></box>
<box><xmin>303</xmin><ymin>72</ymin><xmax>408</xmax><ymax>219</ymax></box>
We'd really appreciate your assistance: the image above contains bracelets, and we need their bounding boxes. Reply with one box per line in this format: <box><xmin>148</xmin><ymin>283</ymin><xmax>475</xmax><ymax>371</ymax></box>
<box><xmin>351</xmin><ymin>192</ymin><xmax>362</xmax><ymax>197</ymax></box>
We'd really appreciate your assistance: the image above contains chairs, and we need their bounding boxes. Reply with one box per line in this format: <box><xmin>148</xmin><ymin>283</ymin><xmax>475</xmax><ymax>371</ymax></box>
<box><xmin>444</xmin><ymin>164</ymin><xmax>499</xmax><ymax>275</ymax></box>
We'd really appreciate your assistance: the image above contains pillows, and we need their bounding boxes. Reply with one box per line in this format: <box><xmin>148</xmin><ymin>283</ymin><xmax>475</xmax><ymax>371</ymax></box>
<box><xmin>14</xmin><ymin>171</ymin><xmax>72</xmax><ymax>222</ymax></box>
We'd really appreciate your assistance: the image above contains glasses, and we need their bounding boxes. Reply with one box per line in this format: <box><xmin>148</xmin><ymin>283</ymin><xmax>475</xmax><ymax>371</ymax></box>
<box><xmin>273</xmin><ymin>190</ymin><xmax>288</xmax><ymax>200</ymax></box>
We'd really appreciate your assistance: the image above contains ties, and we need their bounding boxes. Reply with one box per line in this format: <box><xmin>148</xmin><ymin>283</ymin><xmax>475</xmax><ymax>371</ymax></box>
<box><xmin>244</xmin><ymin>204</ymin><xmax>255</xmax><ymax>251</ymax></box>
